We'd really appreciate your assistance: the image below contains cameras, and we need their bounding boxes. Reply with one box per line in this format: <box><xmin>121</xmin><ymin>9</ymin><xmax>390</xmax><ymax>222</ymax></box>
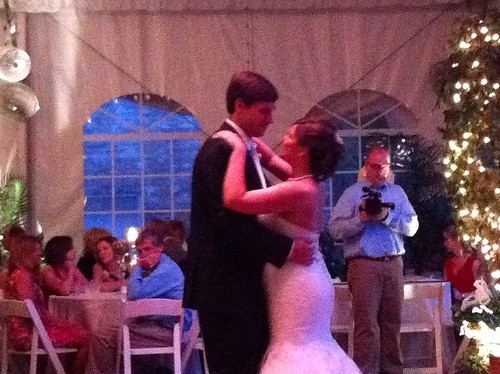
<box><xmin>362</xmin><ymin>186</ymin><xmax>395</xmax><ymax>214</ymax></box>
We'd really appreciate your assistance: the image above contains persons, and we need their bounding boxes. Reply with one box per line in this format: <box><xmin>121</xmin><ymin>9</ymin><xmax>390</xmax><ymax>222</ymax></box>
<box><xmin>93</xmin><ymin>237</ymin><xmax>128</xmax><ymax>292</ymax></box>
<box><xmin>124</xmin><ymin>230</ymin><xmax>185</xmax><ymax>348</ymax></box>
<box><xmin>443</xmin><ymin>225</ymin><xmax>481</xmax><ymax>298</ymax></box>
<box><xmin>328</xmin><ymin>147</ymin><xmax>419</xmax><ymax>374</ymax></box>
<box><xmin>181</xmin><ymin>71</ymin><xmax>317</xmax><ymax>374</ymax></box>
<box><xmin>76</xmin><ymin>218</ymin><xmax>189</xmax><ymax>281</ymax></box>
<box><xmin>212</xmin><ymin>121</ymin><xmax>362</xmax><ymax>374</ymax></box>
<box><xmin>4</xmin><ymin>236</ymin><xmax>114</xmax><ymax>374</ymax></box>
<box><xmin>40</xmin><ymin>236</ymin><xmax>90</xmax><ymax>300</ymax></box>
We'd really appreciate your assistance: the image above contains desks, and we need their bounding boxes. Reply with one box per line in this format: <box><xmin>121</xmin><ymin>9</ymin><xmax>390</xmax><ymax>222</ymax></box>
<box><xmin>44</xmin><ymin>292</ymin><xmax>128</xmax><ymax>374</ymax></box>
<box><xmin>330</xmin><ymin>276</ymin><xmax>457</xmax><ymax>374</ymax></box>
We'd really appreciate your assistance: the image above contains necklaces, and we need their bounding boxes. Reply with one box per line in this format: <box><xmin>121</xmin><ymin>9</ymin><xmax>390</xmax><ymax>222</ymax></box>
<box><xmin>287</xmin><ymin>175</ymin><xmax>314</xmax><ymax>180</ymax></box>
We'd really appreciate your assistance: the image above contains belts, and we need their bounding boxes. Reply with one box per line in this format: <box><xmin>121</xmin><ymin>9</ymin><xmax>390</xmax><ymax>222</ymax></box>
<box><xmin>353</xmin><ymin>254</ymin><xmax>395</xmax><ymax>261</ymax></box>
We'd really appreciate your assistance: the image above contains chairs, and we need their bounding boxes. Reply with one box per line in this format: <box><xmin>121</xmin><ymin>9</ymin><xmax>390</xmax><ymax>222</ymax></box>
<box><xmin>0</xmin><ymin>289</ymin><xmax>78</xmax><ymax>374</ymax></box>
<box><xmin>348</xmin><ymin>283</ymin><xmax>445</xmax><ymax>374</ymax></box>
<box><xmin>116</xmin><ymin>298</ymin><xmax>210</xmax><ymax>374</ymax></box>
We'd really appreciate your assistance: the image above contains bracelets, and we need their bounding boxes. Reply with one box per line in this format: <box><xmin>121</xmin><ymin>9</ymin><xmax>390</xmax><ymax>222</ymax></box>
<box><xmin>380</xmin><ymin>212</ymin><xmax>389</xmax><ymax>221</ymax></box>
